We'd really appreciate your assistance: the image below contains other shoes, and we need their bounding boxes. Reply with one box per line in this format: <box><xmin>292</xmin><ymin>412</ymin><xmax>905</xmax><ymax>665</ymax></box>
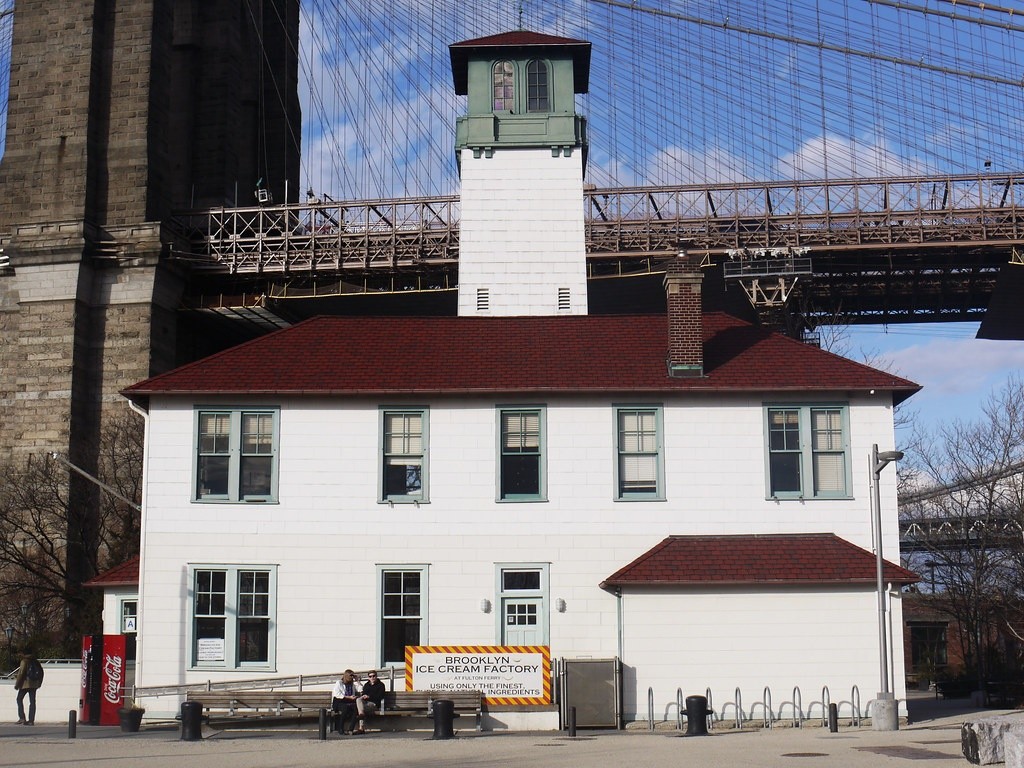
<box><xmin>353</xmin><ymin>715</ymin><xmax>367</xmax><ymax>721</ymax></box>
<box><xmin>353</xmin><ymin>730</ymin><xmax>365</xmax><ymax>735</ymax></box>
<box><xmin>15</xmin><ymin>720</ymin><xmax>25</xmax><ymax>723</ymax></box>
<box><xmin>24</xmin><ymin>722</ymin><xmax>34</xmax><ymax>725</ymax></box>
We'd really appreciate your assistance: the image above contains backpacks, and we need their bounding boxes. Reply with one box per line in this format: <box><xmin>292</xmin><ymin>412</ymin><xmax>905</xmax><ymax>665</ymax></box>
<box><xmin>27</xmin><ymin>658</ymin><xmax>41</xmax><ymax>680</ymax></box>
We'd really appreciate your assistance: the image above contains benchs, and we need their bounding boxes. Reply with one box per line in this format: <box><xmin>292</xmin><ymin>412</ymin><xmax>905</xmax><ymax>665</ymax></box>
<box><xmin>329</xmin><ymin>690</ymin><xmax>483</xmax><ymax>734</ymax></box>
<box><xmin>186</xmin><ymin>691</ymin><xmax>332</xmax><ymax>734</ymax></box>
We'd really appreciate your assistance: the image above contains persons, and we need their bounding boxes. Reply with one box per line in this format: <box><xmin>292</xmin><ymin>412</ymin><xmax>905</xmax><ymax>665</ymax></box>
<box><xmin>353</xmin><ymin>670</ymin><xmax>386</xmax><ymax>735</ymax></box>
<box><xmin>330</xmin><ymin>669</ymin><xmax>358</xmax><ymax>735</ymax></box>
<box><xmin>14</xmin><ymin>646</ymin><xmax>44</xmax><ymax>725</ymax></box>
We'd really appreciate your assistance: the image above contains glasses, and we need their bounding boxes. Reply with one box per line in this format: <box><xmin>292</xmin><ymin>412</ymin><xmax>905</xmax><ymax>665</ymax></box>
<box><xmin>369</xmin><ymin>675</ymin><xmax>375</xmax><ymax>678</ymax></box>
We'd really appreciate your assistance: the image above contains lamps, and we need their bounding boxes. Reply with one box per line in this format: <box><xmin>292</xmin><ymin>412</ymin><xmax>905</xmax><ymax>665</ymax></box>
<box><xmin>481</xmin><ymin>599</ymin><xmax>491</xmax><ymax>614</ymax></box>
<box><xmin>556</xmin><ymin>597</ymin><xmax>565</xmax><ymax>613</ymax></box>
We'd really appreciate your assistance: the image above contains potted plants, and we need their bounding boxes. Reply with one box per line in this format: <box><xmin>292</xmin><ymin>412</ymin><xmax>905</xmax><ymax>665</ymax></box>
<box><xmin>117</xmin><ymin>697</ymin><xmax>145</xmax><ymax>732</ymax></box>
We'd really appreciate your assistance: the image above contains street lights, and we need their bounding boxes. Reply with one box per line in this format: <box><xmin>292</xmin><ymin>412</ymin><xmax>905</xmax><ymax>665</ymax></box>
<box><xmin>22</xmin><ymin>602</ymin><xmax>28</xmax><ymax>649</ymax></box>
<box><xmin>65</xmin><ymin>607</ymin><xmax>73</xmax><ymax>624</ymax></box>
<box><xmin>871</xmin><ymin>444</ymin><xmax>904</xmax><ymax>730</ymax></box>
<box><xmin>4</xmin><ymin>624</ymin><xmax>14</xmax><ymax>672</ymax></box>
<box><xmin>984</xmin><ymin>160</ymin><xmax>993</xmax><ymax>208</ymax></box>
<box><xmin>925</xmin><ymin>561</ymin><xmax>991</xmax><ymax>708</ymax></box>
